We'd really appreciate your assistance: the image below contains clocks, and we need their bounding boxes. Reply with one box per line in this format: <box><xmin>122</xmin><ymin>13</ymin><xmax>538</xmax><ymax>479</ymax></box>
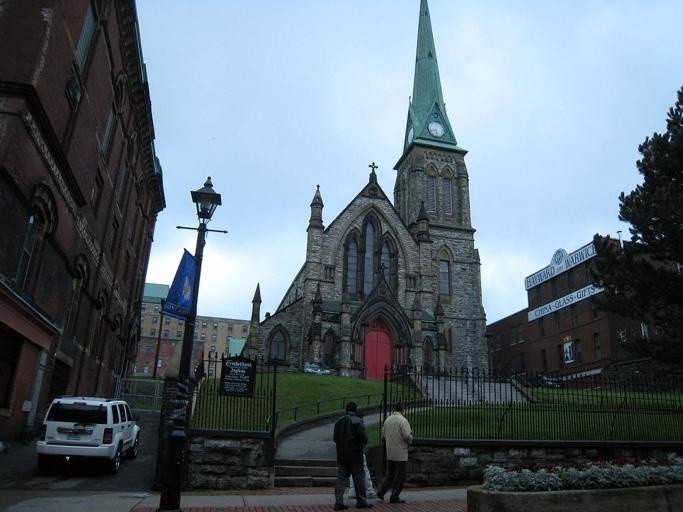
<box><xmin>427</xmin><ymin>121</ymin><xmax>445</xmax><ymax>137</ymax></box>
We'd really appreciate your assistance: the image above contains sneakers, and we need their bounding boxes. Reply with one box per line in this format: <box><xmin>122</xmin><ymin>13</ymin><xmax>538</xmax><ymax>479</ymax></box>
<box><xmin>355</xmin><ymin>501</ymin><xmax>374</xmax><ymax>509</ymax></box>
<box><xmin>389</xmin><ymin>498</ymin><xmax>405</xmax><ymax>504</ymax></box>
<box><xmin>347</xmin><ymin>493</ymin><xmax>387</xmax><ymax>503</ymax></box>
<box><xmin>334</xmin><ymin>503</ymin><xmax>349</xmax><ymax>511</ymax></box>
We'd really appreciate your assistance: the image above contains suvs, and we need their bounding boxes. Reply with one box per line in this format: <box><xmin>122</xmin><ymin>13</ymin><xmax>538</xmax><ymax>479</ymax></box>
<box><xmin>525</xmin><ymin>372</ymin><xmax>564</xmax><ymax>389</ymax></box>
<box><xmin>34</xmin><ymin>394</ymin><xmax>142</xmax><ymax>477</ymax></box>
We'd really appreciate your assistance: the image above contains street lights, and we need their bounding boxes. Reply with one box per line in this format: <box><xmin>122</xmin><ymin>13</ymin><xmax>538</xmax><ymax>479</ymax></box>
<box><xmin>152</xmin><ymin>176</ymin><xmax>229</xmax><ymax>511</ymax></box>
<box><xmin>151</xmin><ymin>297</ymin><xmax>165</xmax><ymax>378</ymax></box>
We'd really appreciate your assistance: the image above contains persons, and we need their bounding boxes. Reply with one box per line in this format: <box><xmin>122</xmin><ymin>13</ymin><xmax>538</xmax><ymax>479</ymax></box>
<box><xmin>332</xmin><ymin>401</ymin><xmax>374</xmax><ymax>510</ymax></box>
<box><xmin>348</xmin><ymin>409</ymin><xmax>380</xmax><ymax>499</ymax></box>
<box><xmin>374</xmin><ymin>403</ymin><xmax>415</xmax><ymax>504</ymax></box>
